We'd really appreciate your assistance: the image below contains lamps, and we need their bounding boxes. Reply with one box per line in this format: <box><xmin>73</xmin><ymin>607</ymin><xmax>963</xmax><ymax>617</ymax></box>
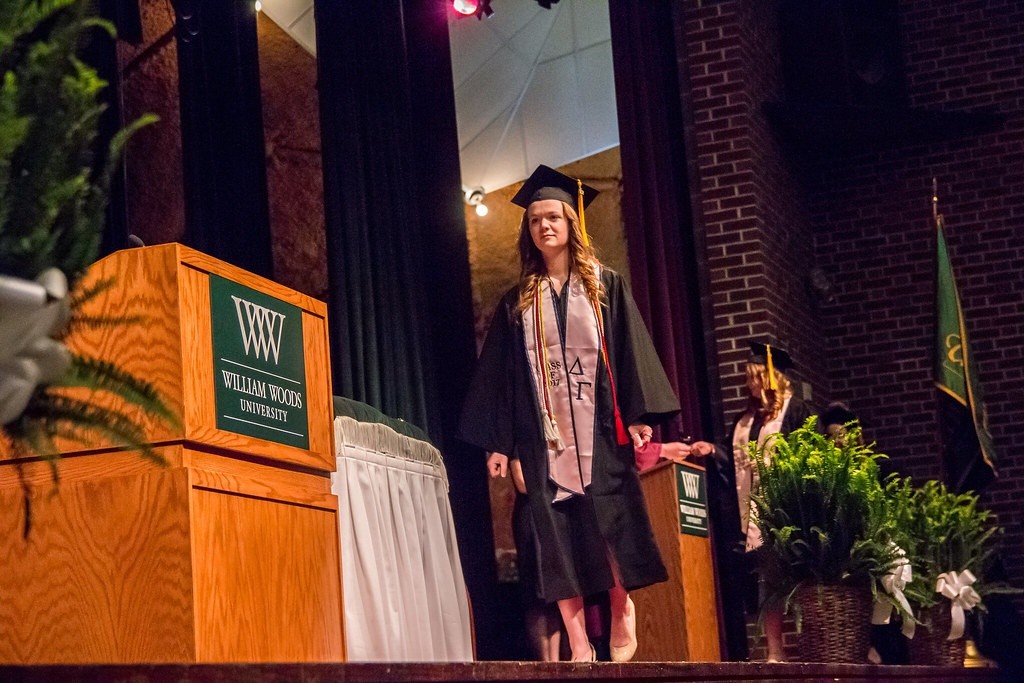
<box><xmin>461</xmin><ymin>184</ymin><xmax>488</xmax><ymax>215</ymax></box>
<box><xmin>450</xmin><ymin>0</ymin><xmax>482</xmax><ymax>19</ymax></box>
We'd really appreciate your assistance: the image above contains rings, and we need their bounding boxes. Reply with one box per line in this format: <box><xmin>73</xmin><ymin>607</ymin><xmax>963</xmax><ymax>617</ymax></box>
<box><xmin>643</xmin><ymin>434</ymin><xmax>652</xmax><ymax>437</ymax></box>
<box><xmin>631</xmin><ymin>434</ymin><xmax>638</xmax><ymax>437</ymax></box>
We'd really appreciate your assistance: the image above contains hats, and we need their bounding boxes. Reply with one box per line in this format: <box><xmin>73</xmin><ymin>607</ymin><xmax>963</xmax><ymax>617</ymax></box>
<box><xmin>510</xmin><ymin>164</ymin><xmax>601</xmax><ymax>248</ymax></box>
<box><xmin>819</xmin><ymin>405</ymin><xmax>865</xmax><ymax>450</ymax></box>
<box><xmin>745</xmin><ymin>338</ymin><xmax>795</xmax><ymax>390</ymax></box>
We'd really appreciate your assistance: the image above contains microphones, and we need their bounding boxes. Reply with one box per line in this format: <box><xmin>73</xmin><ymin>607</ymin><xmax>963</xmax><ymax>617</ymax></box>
<box><xmin>128</xmin><ymin>234</ymin><xmax>145</xmax><ymax>248</ymax></box>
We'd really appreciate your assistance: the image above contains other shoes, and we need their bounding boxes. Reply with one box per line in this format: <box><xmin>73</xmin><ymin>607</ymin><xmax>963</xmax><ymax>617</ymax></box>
<box><xmin>609</xmin><ymin>598</ymin><xmax>638</xmax><ymax>663</ymax></box>
<box><xmin>589</xmin><ymin>642</ymin><xmax>597</xmax><ymax>661</ymax></box>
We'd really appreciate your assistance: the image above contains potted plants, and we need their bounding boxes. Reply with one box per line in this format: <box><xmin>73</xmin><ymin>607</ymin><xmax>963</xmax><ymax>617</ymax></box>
<box><xmin>879</xmin><ymin>475</ymin><xmax>1003</xmax><ymax>670</ymax></box>
<box><xmin>733</xmin><ymin>414</ymin><xmax>912</xmax><ymax>666</ymax></box>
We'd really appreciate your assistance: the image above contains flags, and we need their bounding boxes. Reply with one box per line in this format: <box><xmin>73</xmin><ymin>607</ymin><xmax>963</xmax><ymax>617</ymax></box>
<box><xmin>936</xmin><ymin>217</ymin><xmax>1000</xmax><ymax>511</ymax></box>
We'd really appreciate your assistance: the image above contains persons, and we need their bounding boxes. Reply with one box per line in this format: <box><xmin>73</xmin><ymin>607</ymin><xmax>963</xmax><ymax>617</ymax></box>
<box><xmin>511</xmin><ymin>441</ymin><xmax>690</xmax><ymax>663</ymax></box>
<box><xmin>691</xmin><ymin>340</ymin><xmax>813</xmax><ymax>663</ymax></box>
<box><xmin>823</xmin><ymin>400</ymin><xmax>890</xmax><ymax>664</ymax></box>
<box><xmin>455</xmin><ymin>165</ymin><xmax>683</xmax><ymax>663</ymax></box>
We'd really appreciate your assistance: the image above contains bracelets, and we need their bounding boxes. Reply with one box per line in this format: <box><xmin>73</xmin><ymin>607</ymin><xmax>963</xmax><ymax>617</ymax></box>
<box><xmin>711</xmin><ymin>443</ymin><xmax>715</xmax><ymax>457</ymax></box>
